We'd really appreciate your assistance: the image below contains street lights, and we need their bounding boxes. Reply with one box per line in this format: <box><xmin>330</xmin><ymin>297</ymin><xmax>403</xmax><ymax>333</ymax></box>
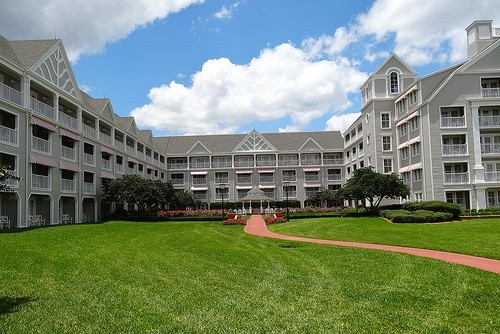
<box><xmin>219</xmin><ymin>183</ymin><xmax>227</xmax><ymax>221</ymax></box>
<box><xmin>282</xmin><ymin>177</ymin><xmax>291</xmax><ymax>222</ymax></box>
